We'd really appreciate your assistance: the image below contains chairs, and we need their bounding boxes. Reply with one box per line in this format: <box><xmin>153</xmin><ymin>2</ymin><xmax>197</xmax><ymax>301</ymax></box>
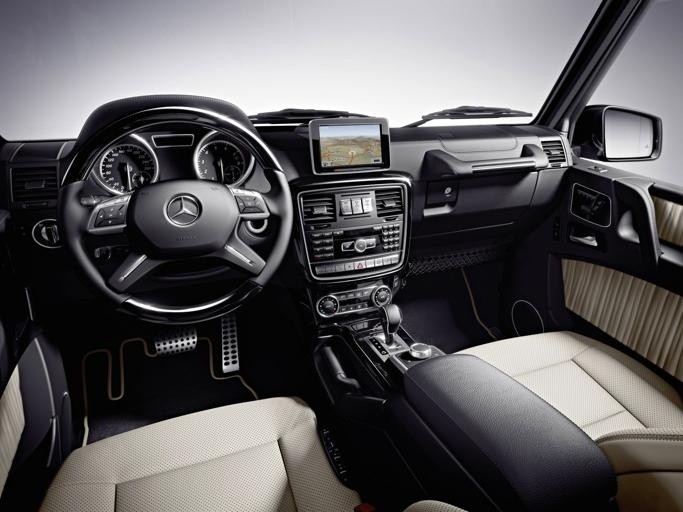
<box><xmin>39</xmin><ymin>391</ymin><xmax>479</xmax><ymax>512</ymax></box>
<box><xmin>403</xmin><ymin>321</ymin><xmax>679</xmax><ymax>511</ymax></box>
